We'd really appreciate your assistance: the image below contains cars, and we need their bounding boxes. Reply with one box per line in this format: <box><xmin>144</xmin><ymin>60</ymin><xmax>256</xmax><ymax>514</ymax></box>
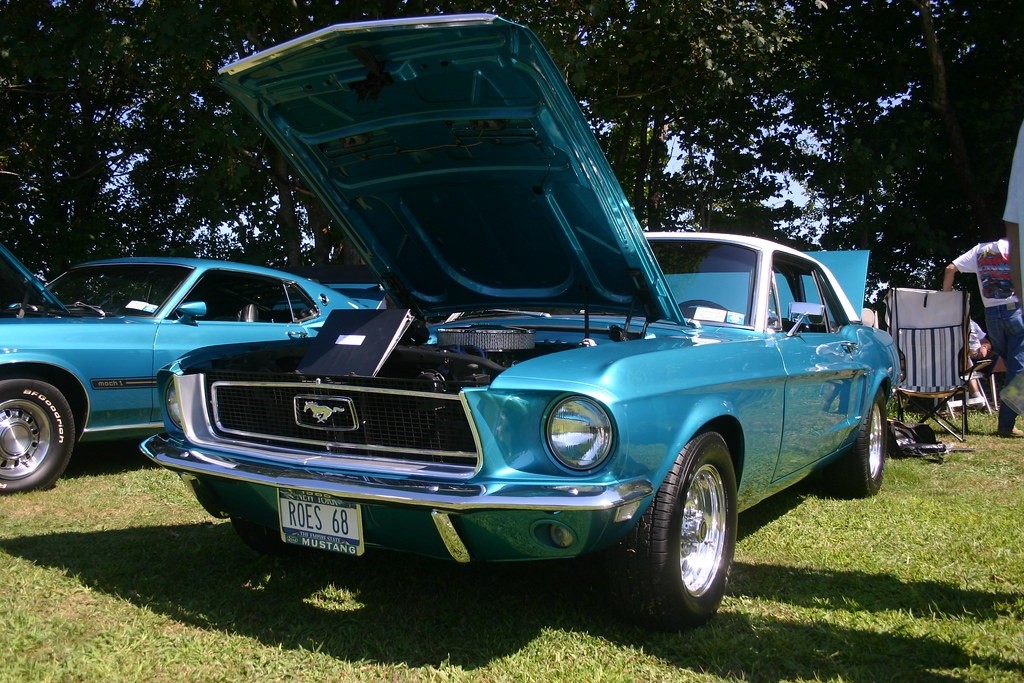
<box><xmin>0</xmin><ymin>244</ymin><xmax>389</xmax><ymax>497</ymax></box>
<box><xmin>139</xmin><ymin>13</ymin><xmax>903</xmax><ymax>632</ymax></box>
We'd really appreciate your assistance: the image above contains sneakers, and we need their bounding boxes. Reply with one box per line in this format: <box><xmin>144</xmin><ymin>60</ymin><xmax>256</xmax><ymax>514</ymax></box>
<box><xmin>972</xmin><ymin>397</ymin><xmax>986</xmax><ymax>410</ymax></box>
<box><xmin>1010</xmin><ymin>426</ymin><xmax>1023</xmax><ymax>437</ymax></box>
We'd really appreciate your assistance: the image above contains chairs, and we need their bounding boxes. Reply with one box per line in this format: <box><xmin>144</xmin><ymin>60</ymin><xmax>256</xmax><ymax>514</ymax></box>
<box><xmin>884</xmin><ymin>287</ymin><xmax>1008</xmax><ymax>441</ymax></box>
<box><xmin>240</xmin><ymin>305</ymin><xmax>260</xmax><ymax>324</ymax></box>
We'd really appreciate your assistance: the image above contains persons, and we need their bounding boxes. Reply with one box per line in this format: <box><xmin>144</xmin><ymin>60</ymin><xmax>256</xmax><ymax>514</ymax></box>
<box><xmin>943</xmin><ymin>238</ymin><xmax>1024</xmax><ymax>437</ymax></box>
<box><xmin>963</xmin><ymin>122</ymin><xmax>1024</xmax><ymax>415</ymax></box>
